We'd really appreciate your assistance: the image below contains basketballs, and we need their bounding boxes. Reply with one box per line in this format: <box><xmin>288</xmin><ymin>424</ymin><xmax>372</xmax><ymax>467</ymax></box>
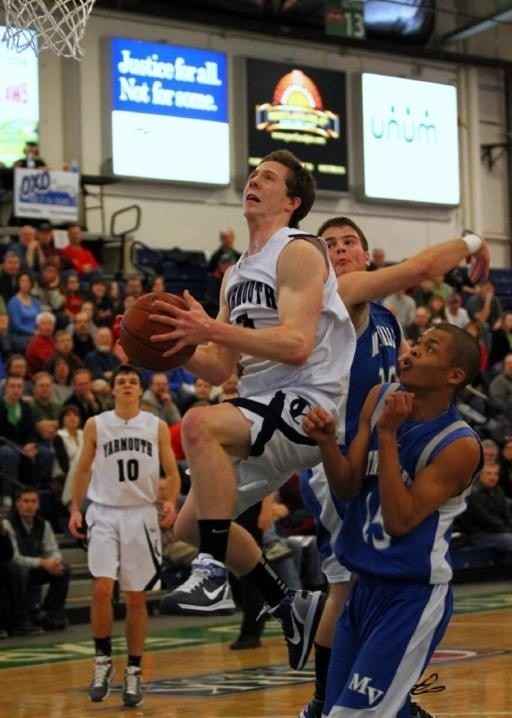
<box><xmin>118</xmin><ymin>293</ymin><xmax>198</xmax><ymax>372</ymax></box>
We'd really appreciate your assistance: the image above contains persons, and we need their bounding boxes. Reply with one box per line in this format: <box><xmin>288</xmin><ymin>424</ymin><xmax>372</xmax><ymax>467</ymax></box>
<box><xmin>369</xmin><ymin>246</ymin><xmax>512</xmax><ymax>555</ymax></box>
<box><xmin>1</xmin><ymin>221</ymin><xmax>242</xmax><ymax>639</ymax></box>
<box><xmin>69</xmin><ymin>368</ymin><xmax>181</xmax><ymax>705</ymax></box>
<box><xmin>147</xmin><ymin>146</ymin><xmax>488</xmax><ymax>716</ymax></box>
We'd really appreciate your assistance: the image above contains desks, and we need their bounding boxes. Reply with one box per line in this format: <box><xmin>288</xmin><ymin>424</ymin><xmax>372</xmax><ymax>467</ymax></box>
<box><xmin>0</xmin><ymin>162</ymin><xmax>120</xmax><ymax>230</ymax></box>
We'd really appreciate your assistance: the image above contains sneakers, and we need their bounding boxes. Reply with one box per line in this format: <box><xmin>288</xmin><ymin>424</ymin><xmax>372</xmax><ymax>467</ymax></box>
<box><xmin>88</xmin><ymin>654</ymin><xmax>115</xmax><ymax>703</ymax></box>
<box><xmin>256</xmin><ymin>587</ymin><xmax>328</xmax><ymax>671</ymax></box>
<box><xmin>157</xmin><ymin>553</ymin><xmax>237</xmax><ymax>617</ymax></box>
<box><xmin>299</xmin><ymin>695</ymin><xmax>325</xmax><ymax>718</ymax></box>
<box><xmin>228</xmin><ymin>631</ymin><xmax>261</xmax><ymax>649</ymax></box>
<box><xmin>397</xmin><ymin>672</ymin><xmax>447</xmax><ymax>717</ymax></box>
<box><xmin>122</xmin><ymin>664</ymin><xmax>144</xmax><ymax>706</ymax></box>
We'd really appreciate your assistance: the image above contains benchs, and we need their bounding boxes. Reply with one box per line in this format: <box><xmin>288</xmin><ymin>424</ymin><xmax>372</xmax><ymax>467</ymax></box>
<box><xmin>40</xmin><ymin>528</ymin><xmax>173</xmax><ymax>615</ymax></box>
